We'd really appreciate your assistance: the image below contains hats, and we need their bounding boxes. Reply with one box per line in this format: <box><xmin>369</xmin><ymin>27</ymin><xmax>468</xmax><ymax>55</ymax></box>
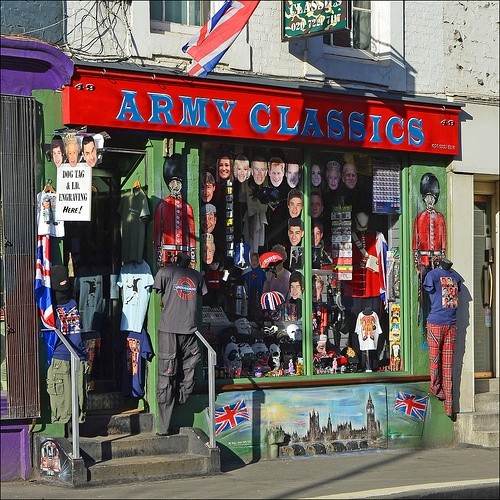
<box><xmin>49</xmin><ymin>265</ymin><xmax>72</xmax><ymax>304</ymax></box>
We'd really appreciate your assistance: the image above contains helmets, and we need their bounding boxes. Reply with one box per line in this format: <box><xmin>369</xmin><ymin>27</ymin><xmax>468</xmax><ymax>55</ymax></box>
<box><xmin>260</xmin><ymin>290</ymin><xmax>286</xmax><ymax>314</ymax></box>
<box><xmin>259</xmin><ymin>252</ymin><xmax>284</xmax><ymax>273</ymax></box>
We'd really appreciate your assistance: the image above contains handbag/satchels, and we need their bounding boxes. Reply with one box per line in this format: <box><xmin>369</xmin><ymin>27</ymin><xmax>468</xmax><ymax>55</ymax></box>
<box><xmin>352</xmin><ymin>232</ymin><xmax>381</xmax><ymax>273</ymax></box>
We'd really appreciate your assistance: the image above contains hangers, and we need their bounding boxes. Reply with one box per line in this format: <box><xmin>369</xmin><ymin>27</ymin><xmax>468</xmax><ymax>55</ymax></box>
<box><xmin>130</xmin><ymin>172</ymin><xmax>143</xmax><ymax>190</ymax></box>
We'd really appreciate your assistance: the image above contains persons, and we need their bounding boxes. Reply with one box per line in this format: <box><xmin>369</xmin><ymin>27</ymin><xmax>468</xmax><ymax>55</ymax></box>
<box><xmin>200</xmin><ymin>233</ymin><xmax>216</xmax><ymax>264</ymax></box>
<box><xmin>82</xmin><ymin>136</ymin><xmax>100</xmax><ymax>168</ymax></box>
<box><xmin>290</xmin><ymin>271</ymin><xmax>306</xmax><ymax>299</ymax></box>
<box><xmin>287</xmin><ymin>190</ymin><xmax>304</xmax><ymax>218</ymax></box>
<box><xmin>338</xmin><ymin>205</ymin><xmax>387</xmax><ymax>368</ymax></box>
<box><xmin>354</xmin><ymin>307</ymin><xmax>383</xmax><ymax>373</ymax></box>
<box><xmin>38</xmin><ymin>264</ymin><xmax>87</xmax><ymax>430</ymax></box>
<box><xmin>150</xmin><ymin>253</ymin><xmax>206</xmax><ymax>437</ymax></box>
<box><xmin>286</xmin><ymin>218</ymin><xmax>305</xmax><ymax>245</ymax></box>
<box><xmin>200</xmin><ymin>171</ymin><xmax>217</xmax><ymax>202</ymax></box>
<box><xmin>424</xmin><ymin>258</ymin><xmax>464</xmax><ymax>418</ymax></box>
<box><xmin>49</xmin><ymin>134</ymin><xmax>66</xmax><ymax>169</ymax></box>
<box><xmin>248</xmin><ymin>252</ymin><xmax>259</xmax><ymax>269</ymax></box>
<box><xmin>214</xmin><ymin>152</ymin><xmax>360</xmax><ymax>190</ymax></box>
<box><xmin>200</xmin><ymin>204</ymin><xmax>218</xmax><ymax>231</ymax></box>
<box><xmin>63</xmin><ymin>133</ymin><xmax>81</xmax><ymax>168</ymax></box>
<box><xmin>313</xmin><ymin>223</ymin><xmax>326</xmax><ymax>247</ymax></box>
<box><xmin>220</xmin><ymin>315</ymin><xmax>341</xmax><ymax>376</ymax></box>
<box><xmin>312</xmin><ymin>192</ymin><xmax>326</xmax><ymax>221</ymax></box>
<box><xmin>312</xmin><ymin>274</ymin><xmax>327</xmax><ymax>304</ymax></box>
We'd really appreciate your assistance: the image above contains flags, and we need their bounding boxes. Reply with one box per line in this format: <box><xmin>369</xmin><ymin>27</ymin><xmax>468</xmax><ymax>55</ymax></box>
<box><xmin>177</xmin><ymin>0</ymin><xmax>262</xmax><ymax>78</ymax></box>
